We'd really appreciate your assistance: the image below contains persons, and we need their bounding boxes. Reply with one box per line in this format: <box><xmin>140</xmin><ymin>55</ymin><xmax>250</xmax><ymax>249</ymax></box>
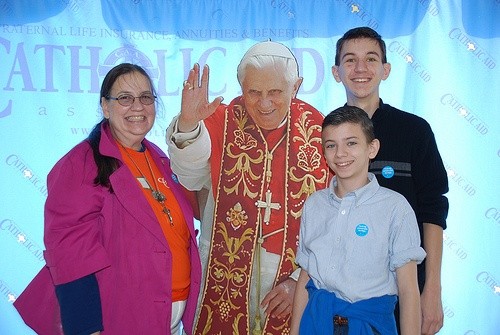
<box><xmin>12</xmin><ymin>63</ymin><xmax>199</xmax><ymax>335</ymax></box>
<box><xmin>165</xmin><ymin>40</ymin><xmax>335</xmax><ymax>335</ymax></box>
<box><xmin>289</xmin><ymin>105</ymin><xmax>423</xmax><ymax>335</ymax></box>
<box><xmin>330</xmin><ymin>26</ymin><xmax>449</xmax><ymax>335</ymax></box>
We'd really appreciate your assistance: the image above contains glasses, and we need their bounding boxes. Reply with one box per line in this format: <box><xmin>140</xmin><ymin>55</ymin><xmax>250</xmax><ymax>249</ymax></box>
<box><xmin>108</xmin><ymin>94</ymin><xmax>158</xmax><ymax>106</ymax></box>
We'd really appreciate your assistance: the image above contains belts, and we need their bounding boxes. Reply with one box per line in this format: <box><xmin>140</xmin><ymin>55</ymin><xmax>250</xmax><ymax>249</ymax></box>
<box><xmin>333</xmin><ymin>315</ymin><xmax>349</xmax><ymax>324</ymax></box>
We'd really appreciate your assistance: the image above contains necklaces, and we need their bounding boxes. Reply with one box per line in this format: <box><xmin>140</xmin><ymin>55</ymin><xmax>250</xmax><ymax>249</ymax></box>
<box><xmin>254</xmin><ymin>132</ymin><xmax>288</xmax><ymax>227</ymax></box>
<box><xmin>111</xmin><ymin>134</ymin><xmax>175</xmax><ymax>227</ymax></box>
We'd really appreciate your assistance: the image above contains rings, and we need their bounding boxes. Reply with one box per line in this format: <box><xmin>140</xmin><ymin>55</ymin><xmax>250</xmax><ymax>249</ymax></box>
<box><xmin>183</xmin><ymin>80</ymin><xmax>194</xmax><ymax>91</ymax></box>
